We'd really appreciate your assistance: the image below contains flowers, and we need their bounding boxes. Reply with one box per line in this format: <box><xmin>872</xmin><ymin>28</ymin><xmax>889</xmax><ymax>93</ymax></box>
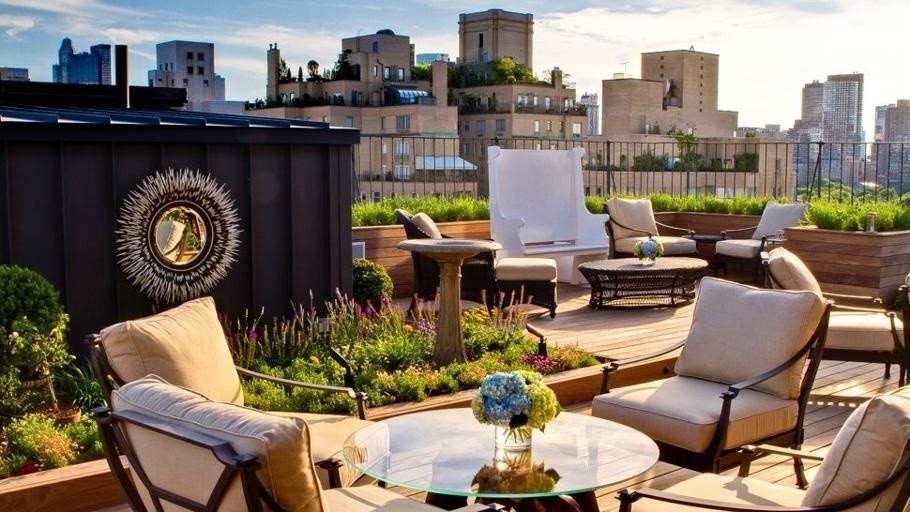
<box><xmin>471</xmin><ymin>368</ymin><xmax>560</xmax><ymax>430</ymax></box>
<box><xmin>634</xmin><ymin>239</ymin><xmax>664</xmax><ymax>257</ymax></box>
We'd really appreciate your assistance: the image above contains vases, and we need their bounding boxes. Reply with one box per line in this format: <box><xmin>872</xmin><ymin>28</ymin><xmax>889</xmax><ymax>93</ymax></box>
<box><xmin>639</xmin><ymin>256</ymin><xmax>655</xmax><ymax>265</ymax></box>
<box><xmin>502</xmin><ymin>420</ymin><xmax>531</xmax><ymax>452</ymax></box>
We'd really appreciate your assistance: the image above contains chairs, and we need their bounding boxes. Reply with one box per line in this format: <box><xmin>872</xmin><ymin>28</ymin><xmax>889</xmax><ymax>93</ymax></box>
<box><xmin>616</xmin><ymin>385</ymin><xmax>910</xmax><ymax>512</ymax></box>
<box><xmin>715</xmin><ymin>200</ymin><xmax>805</xmax><ymax>288</ymax></box>
<box><xmin>605</xmin><ymin>198</ymin><xmax>699</xmax><ymax>289</ymax></box>
<box><xmin>395</xmin><ymin>207</ymin><xmax>499</xmax><ymax>315</ymax></box>
<box><xmin>589</xmin><ymin>275</ymin><xmax>835</xmax><ymax>489</ymax></box>
<box><xmin>759</xmin><ymin>247</ymin><xmax>910</xmax><ymax>386</ymax></box>
<box><xmin>92</xmin><ymin>374</ymin><xmax>446</xmax><ymax>512</ymax></box>
<box><xmin>85</xmin><ymin>297</ymin><xmax>390</xmax><ymax>491</ymax></box>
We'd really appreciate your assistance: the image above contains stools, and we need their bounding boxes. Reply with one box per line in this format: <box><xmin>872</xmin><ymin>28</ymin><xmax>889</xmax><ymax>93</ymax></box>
<box><xmin>496</xmin><ymin>256</ymin><xmax>559</xmax><ymax>319</ymax></box>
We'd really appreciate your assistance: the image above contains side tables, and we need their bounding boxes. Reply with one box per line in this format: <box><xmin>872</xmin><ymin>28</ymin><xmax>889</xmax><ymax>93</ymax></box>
<box><xmin>683</xmin><ymin>234</ymin><xmax>730</xmax><ymax>280</ymax></box>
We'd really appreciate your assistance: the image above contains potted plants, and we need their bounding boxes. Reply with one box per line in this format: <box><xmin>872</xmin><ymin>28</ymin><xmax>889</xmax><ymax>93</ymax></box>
<box><xmin>10</xmin><ymin>313</ymin><xmax>80</xmax><ymax>428</ymax></box>
<box><xmin>2</xmin><ymin>414</ymin><xmax>77</xmax><ymax>475</ymax></box>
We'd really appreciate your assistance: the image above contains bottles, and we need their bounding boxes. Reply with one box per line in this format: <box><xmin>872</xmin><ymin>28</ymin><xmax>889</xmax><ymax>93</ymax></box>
<box><xmin>866</xmin><ymin>213</ymin><xmax>875</xmax><ymax>234</ymax></box>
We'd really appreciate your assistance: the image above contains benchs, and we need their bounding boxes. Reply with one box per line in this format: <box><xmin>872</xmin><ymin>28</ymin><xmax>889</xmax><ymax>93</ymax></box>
<box><xmin>486</xmin><ymin>145</ymin><xmax>609</xmax><ymax>286</ymax></box>
